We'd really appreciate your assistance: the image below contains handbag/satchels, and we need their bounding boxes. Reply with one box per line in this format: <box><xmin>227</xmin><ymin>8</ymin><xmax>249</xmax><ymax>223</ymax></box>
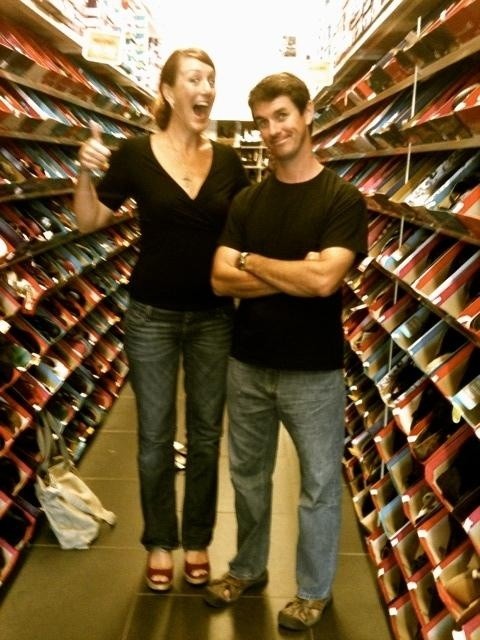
<box><xmin>38</xmin><ymin>460</ymin><xmax>115</xmax><ymax>551</ymax></box>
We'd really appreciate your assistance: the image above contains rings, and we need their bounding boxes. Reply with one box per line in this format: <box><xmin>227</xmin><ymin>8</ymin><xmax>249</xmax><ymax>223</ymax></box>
<box><xmin>96</xmin><ymin>153</ymin><xmax>100</xmax><ymax>159</ymax></box>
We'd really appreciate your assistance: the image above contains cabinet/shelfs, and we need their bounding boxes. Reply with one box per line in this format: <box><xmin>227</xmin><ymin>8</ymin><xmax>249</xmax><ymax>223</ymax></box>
<box><xmin>0</xmin><ymin>0</ymin><xmax>157</xmax><ymax>602</ymax></box>
<box><xmin>314</xmin><ymin>3</ymin><xmax>480</xmax><ymax>640</ymax></box>
<box><xmin>217</xmin><ymin>122</ymin><xmax>272</xmax><ymax>181</ymax></box>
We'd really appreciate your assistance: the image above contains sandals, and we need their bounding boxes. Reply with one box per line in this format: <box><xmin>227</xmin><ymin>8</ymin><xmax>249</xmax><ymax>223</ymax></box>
<box><xmin>183</xmin><ymin>561</ymin><xmax>210</xmax><ymax>586</ymax></box>
<box><xmin>203</xmin><ymin>568</ymin><xmax>268</xmax><ymax>607</ymax></box>
<box><xmin>145</xmin><ymin>566</ymin><xmax>174</xmax><ymax>592</ymax></box>
<box><xmin>278</xmin><ymin>594</ymin><xmax>333</xmax><ymax>631</ymax></box>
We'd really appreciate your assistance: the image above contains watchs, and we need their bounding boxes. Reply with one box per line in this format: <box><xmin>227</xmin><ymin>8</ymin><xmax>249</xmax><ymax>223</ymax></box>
<box><xmin>233</xmin><ymin>250</ymin><xmax>251</xmax><ymax>270</ymax></box>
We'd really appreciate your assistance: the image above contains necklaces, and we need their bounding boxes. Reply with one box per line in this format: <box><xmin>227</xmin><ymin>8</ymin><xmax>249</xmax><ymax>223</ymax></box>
<box><xmin>166</xmin><ymin>129</ymin><xmax>200</xmax><ymax>189</ymax></box>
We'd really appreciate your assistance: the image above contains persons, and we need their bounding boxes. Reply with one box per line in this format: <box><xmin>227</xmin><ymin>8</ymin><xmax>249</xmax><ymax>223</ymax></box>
<box><xmin>206</xmin><ymin>73</ymin><xmax>369</xmax><ymax>633</ymax></box>
<box><xmin>74</xmin><ymin>50</ymin><xmax>254</xmax><ymax>593</ymax></box>
